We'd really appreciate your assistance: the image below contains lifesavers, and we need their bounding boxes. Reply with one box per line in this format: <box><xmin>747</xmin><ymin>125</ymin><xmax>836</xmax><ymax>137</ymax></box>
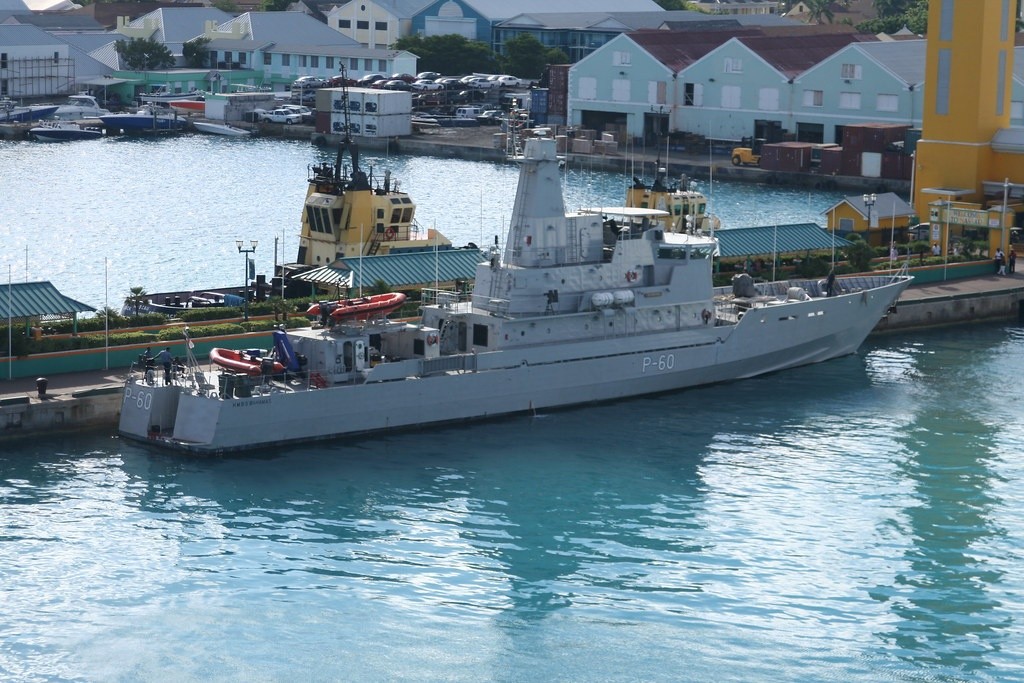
<box><xmin>146</xmin><ymin>369</ymin><xmax>155</xmax><ymax>386</ymax></box>
<box><xmin>817</xmin><ymin>279</ymin><xmax>828</xmax><ymax>296</ymax></box>
<box><xmin>427</xmin><ymin>332</ymin><xmax>438</xmax><ymax>347</ymax></box>
<box><xmin>627</xmin><ymin>269</ymin><xmax>638</xmax><ymax>283</ymax></box>
<box><xmin>702</xmin><ymin>308</ymin><xmax>712</xmax><ymax>321</ymax></box>
<box><xmin>386</xmin><ymin>228</ymin><xmax>395</xmax><ymax>239</ymax></box>
<box><xmin>657</xmin><ymin>245</ymin><xmax>666</xmax><ymax>256</ymax></box>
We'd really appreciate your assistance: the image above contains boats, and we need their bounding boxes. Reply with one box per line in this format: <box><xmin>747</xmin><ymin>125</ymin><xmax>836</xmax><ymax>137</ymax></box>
<box><xmin>210</xmin><ymin>348</ymin><xmax>284</xmax><ymax>375</ymax></box>
<box><xmin>119</xmin><ymin>126</ymin><xmax>915</xmax><ymax>455</ymax></box>
<box><xmin>54</xmin><ymin>95</ymin><xmax>111</xmax><ymax>119</ymax></box>
<box><xmin>306</xmin><ymin>292</ymin><xmax>407</xmax><ymax>321</ymax></box>
<box><xmin>139</xmin><ymin>87</ymin><xmax>200</xmax><ymax>102</ymax></box>
<box><xmin>167</xmin><ymin>100</ymin><xmax>206</xmax><ymax>113</ymax></box>
<box><xmin>0</xmin><ymin>97</ymin><xmax>59</xmax><ymax>122</ymax></box>
<box><xmin>193</xmin><ymin>121</ymin><xmax>251</xmax><ymax>136</ymax></box>
<box><xmin>120</xmin><ymin>61</ymin><xmax>479</xmax><ymax>319</ymax></box>
<box><xmin>99</xmin><ymin>102</ymin><xmax>186</xmax><ymax>129</ymax></box>
<box><xmin>626</xmin><ymin>168</ymin><xmax>720</xmax><ymax>232</ymax></box>
<box><xmin>29</xmin><ymin>116</ymin><xmax>101</xmax><ymax>141</ymax></box>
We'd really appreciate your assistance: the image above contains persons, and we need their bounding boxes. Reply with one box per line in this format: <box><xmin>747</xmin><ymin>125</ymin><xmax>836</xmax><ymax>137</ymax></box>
<box><xmin>735</xmin><ymin>259</ymin><xmax>774</xmax><ymax>273</ymax></box>
<box><xmin>172</xmin><ymin>357</ymin><xmax>185</xmax><ymax>380</ymax></box>
<box><xmin>995</xmin><ymin>248</ymin><xmax>1006</xmax><ymax>276</ymax></box>
<box><xmin>826</xmin><ymin>270</ymin><xmax>835</xmax><ymax>297</ymax></box>
<box><xmin>642</xmin><ymin>216</ymin><xmax>649</xmax><ymax>232</ymax></box>
<box><xmin>892</xmin><ymin>247</ymin><xmax>898</xmax><ymax>261</ymax></box>
<box><xmin>544</xmin><ymin>290</ymin><xmax>559</xmax><ymax>315</ymax></box>
<box><xmin>1009</xmin><ymin>250</ymin><xmax>1016</xmax><ymax>273</ymax></box>
<box><xmin>144</xmin><ymin>347</ymin><xmax>155</xmax><ymax>381</ymax></box>
<box><xmin>932</xmin><ymin>243</ymin><xmax>941</xmax><ymax>256</ymax></box>
<box><xmin>151</xmin><ymin>347</ymin><xmax>176</xmax><ymax>386</ymax></box>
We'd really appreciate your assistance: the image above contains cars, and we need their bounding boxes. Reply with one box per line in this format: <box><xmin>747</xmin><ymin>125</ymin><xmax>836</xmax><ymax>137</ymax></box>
<box><xmin>908</xmin><ymin>223</ymin><xmax>930</xmax><ymax>235</ymax></box>
<box><xmin>296</xmin><ymin>90</ymin><xmax>316</xmax><ymax>102</ymax></box>
<box><xmin>382</xmin><ymin>80</ymin><xmax>413</xmax><ymax>92</ymax></box>
<box><xmin>358</xmin><ymin>74</ymin><xmax>387</xmax><ymax>87</ymax></box>
<box><xmin>281</xmin><ymin>104</ymin><xmax>312</xmax><ymax>116</ymax></box>
<box><xmin>409</xmin><ymin>80</ymin><xmax>444</xmax><ymax>91</ymax></box>
<box><xmin>477</xmin><ymin>111</ymin><xmax>506</xmax><ymax>124</ymax></box>
<box><xmin>370</xmin><ymin>80</ymin><xmax>389</xmax><ymax>89</ymax></box>
<box><xmin>388</xmin><ymin>73</ymin><xmax>415</xmax><ymax>83</ymax></box>
<box><xmin>456</xmin><ymin>108</ymin><xmax>484</xmax><ymax>117</ymax></box>
<box><xmin>458</xmin><ymin>74</ymin><xmax>522</xmax><ymax>89</ymax></box>
<box><xmin>327</xmin><ymin>76</ymin><xmax>358</xmax><ymax>87</ymax></box>
<box><xmin>434</xmin><ymin>78</ymin><xmax>466</xmax><ymax>90</ymax></box>
<box><xmin>294</xmin><ymin>76</ymin><xmax>328</xmax><ymax>88</ymax></box>
<box><xmin>415</xmin><ymin>72</ymin><xmax>442</xmax><ymax>81</ymax></box>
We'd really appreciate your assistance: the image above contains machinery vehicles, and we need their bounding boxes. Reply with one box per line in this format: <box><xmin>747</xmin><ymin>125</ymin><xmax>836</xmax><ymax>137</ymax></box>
<box><xmin>732</xmin><ymin>136</ymin><xmax>767</xmax><ymax>165</ymax></box>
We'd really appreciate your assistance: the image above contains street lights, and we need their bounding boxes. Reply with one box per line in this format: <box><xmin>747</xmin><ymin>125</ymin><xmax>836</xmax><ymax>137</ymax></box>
<box><xmin>236</xmin><ymin>240</ymin><xmax>258</xmax><ymax>321</ymax></box>
<box><xmin>910</xmin><ymin>151</ymin><xmax>915</xmax><ymax>208</ymax></box>
<box><xmin>527</xmin><ymin>98</ymin><xmax>532</xmax><ymax>128</ymax></box>
<box><xmin>863</xmin><ymin>194</ymin><xmax>876</xmax><ymax>243</ymax></box>
<box><xmin>1000</xmin><ymin>178</ymin><xmax>1013</xmax><ymax>252</ymax></box>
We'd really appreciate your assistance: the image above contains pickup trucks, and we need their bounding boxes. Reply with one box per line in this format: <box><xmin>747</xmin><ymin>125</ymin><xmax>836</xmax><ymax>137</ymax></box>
<box><xmin>261</xmin><ymin>109</ymin><xmax>302</xmax><ymax>125</ymax></box>
